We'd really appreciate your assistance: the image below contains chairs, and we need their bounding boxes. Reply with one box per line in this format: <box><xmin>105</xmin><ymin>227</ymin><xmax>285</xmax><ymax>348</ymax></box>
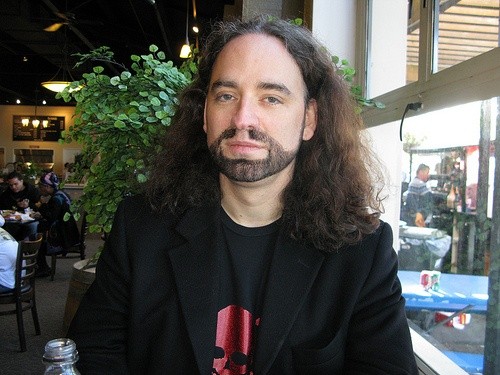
<box><xmin>50</xmin><ymin>211</ymin><xmax>87</xmax><ymax>281</ymax></box>
<box><xmin>0</xmin><ymin>235</ymin><xmax>43</xmax><ymax>353</ymax></box>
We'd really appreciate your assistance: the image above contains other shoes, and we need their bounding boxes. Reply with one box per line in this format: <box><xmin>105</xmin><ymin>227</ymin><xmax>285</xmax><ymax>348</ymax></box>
<box><xmin>34</xmin><ymin>267</ymin><xmax>52</xmax><ymax>279</ymax></box>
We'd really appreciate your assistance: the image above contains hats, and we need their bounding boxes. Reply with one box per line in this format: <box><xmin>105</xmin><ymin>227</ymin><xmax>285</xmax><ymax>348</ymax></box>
<box><xmin>39</xmin><ymin>171</ymin><xmax>60</xmax><ymax>188</ymax></box>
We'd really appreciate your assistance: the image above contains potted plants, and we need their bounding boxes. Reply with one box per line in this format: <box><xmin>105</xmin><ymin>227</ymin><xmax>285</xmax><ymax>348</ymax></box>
<box><xmin>55</xmin><ymin>13</ymin><xmax>387</xmax><ymax>332</ymax></box>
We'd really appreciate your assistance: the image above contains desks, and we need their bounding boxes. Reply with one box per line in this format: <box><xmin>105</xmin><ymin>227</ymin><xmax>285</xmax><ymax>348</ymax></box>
<box><xmin>398</xmin><ymin>270</ymin><xmax>489</xmax><ymax>335</ymax></box>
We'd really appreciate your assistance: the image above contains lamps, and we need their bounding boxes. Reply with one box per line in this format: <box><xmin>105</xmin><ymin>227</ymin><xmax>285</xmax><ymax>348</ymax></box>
<box><xmin>21</xmin><ymin>106</ymin><xmax>51</xmax><ymax>129</ymax></box>
<box><xmin>41</xmin><ymin>26</ymin><xmax>85</xmax><ymax>93</ymax></box>
<box><xmin>42</xmin><ymin>9</ymin><xmax>80</xmax><ymax>33</ymax></box>
<box><xmin>179</xmin><ymin>0</ymin><xmax>192</xmax><ymax>58</ymax></box>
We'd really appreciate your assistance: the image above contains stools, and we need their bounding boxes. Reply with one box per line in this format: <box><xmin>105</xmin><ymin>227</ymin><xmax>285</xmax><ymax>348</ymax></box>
<box><xmin>442</xmin><ymin>351</ymin><xmax>484</xmax><ymax>375</ymax></box>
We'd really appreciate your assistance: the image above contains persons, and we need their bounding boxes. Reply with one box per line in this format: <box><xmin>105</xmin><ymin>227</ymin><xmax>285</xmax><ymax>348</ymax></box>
<box><xmin>0</xmin><ymin>214</ymin><xmax>28</xmax><ymax>294</ymax></box>
<box><xmin>31</xmin><ymin>171</ymin><xmax>79</xmax><ymax>278</ymax></box>
<box><xmin>405</xmin><ymin>164</ymin><xmax>434</xmax><ymax>227</ymax></box>
<box><xmin>68</xmin><ymin>15</ymin><xmax>418</xmax><ymax>375</ymax></box>
<box><xmin>0</xmin><ymin>172</ymin><xmax>43</xmax><ymax>242</ymax></box>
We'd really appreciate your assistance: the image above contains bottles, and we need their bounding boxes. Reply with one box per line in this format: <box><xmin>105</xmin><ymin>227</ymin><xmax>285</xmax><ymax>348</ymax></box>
<box><xmin>41</xmin><ymin>338</ymin><xmax>80</xmax><ymax>375</ymax></box>
<box><xmin>446</xmin><ymin>184</ymin><xmax>456</xmax><ymax>208</ymax></box>
<box><xmin>453</xmin><ymin>186</ymin><xmax>462</xmax><ymax>213</ymax></box>
